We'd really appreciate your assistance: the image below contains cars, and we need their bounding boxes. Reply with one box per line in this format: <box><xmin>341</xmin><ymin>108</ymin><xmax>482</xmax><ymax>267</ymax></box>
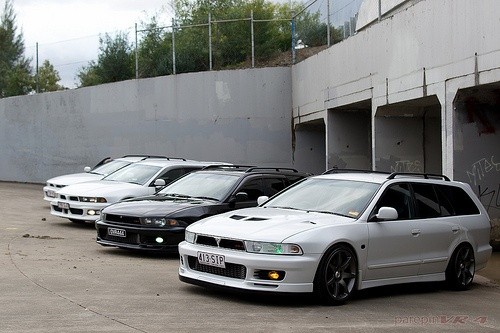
<box><xmin>95</xmin><ymin>164</ymin><xmax>314</xmax><ymax>257</ymax></box>
<box><xmin>50</xmin><ymin>155</ymin><xmax>235</xmax><ymax>224</ymax></box>
<box><xmin>178</xmin><ymin>169</ymin><xmax>493</xmax><ymax>306</ymax></box>
<box><xmin>43</xmin><ymin>155</ymin><xmax>165</xmax><ymax>202</ymax></box>
